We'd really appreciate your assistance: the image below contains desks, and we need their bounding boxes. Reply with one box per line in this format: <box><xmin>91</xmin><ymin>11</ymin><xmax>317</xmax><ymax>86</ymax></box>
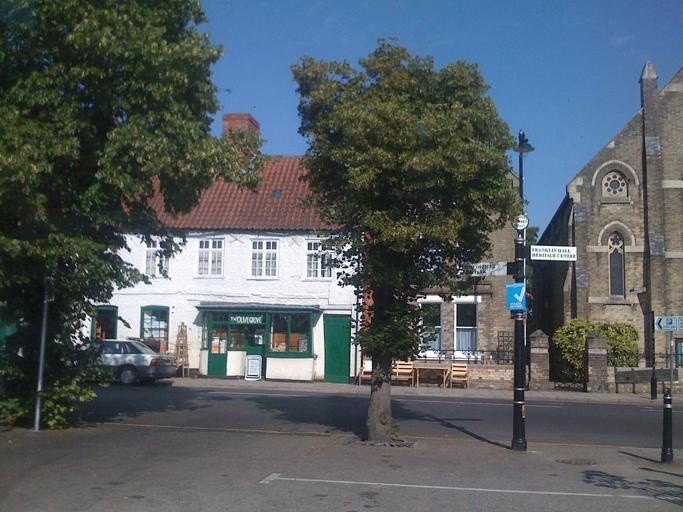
<box><xmin>412</xmin><ymin>361</ymin><xmax>451</xmax><ymax>389</ymax></box>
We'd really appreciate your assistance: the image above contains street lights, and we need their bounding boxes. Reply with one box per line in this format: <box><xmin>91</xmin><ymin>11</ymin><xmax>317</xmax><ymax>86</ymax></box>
<box><xmin>509</xmin><ymin>127</ymin><xmax>536</xmax><ymax>454</ymax></box>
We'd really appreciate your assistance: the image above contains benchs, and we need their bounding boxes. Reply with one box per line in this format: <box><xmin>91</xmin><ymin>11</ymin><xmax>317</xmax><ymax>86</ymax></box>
<box><xmin>358</xmin><ymin>360</ymin><xmax>415</xmax><ymax>385</ymax></box>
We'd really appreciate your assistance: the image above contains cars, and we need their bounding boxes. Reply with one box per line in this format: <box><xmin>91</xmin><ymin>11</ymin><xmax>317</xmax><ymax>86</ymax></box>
<box><xmin>82</xmin><ymin>339</ymin><xmax>175</xmax><ymax>388</ymax></box>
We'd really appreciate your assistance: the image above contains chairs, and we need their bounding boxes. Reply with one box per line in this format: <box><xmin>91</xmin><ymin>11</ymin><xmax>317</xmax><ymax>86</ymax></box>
<box><xmin>448</xmin><ymin>362</ymin><xmax>469</xmax><ymax>389</ymax></box>
